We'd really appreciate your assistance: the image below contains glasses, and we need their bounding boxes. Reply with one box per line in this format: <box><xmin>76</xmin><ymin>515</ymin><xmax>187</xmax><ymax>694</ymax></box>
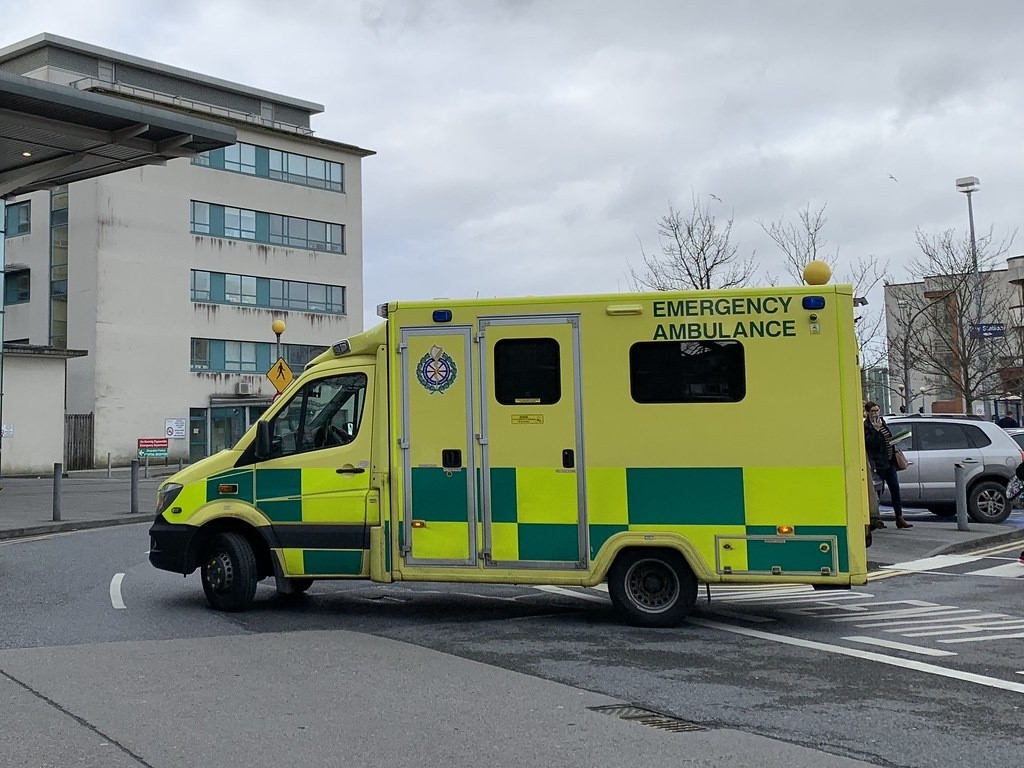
<box><xmin>869</xmin><ymin>409</ymin><xmax>881</xmax><ymax>413</ymax></box>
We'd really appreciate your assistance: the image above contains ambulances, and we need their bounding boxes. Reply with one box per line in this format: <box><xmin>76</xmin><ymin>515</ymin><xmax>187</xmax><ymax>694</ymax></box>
<box><xmin>148</xmin><ymin>284</ymin><xmax>873</xmax><ymax>629</ymax></box>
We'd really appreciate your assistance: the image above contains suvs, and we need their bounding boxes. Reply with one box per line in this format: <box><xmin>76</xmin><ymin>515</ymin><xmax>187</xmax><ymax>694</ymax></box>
<box><xmin>864</xmin><ymin>414</ymin><xmax>1024</xmax><ymax>524</ymax></box>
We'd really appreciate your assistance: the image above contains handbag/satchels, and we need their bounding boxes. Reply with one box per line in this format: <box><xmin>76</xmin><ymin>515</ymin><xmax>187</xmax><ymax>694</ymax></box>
<box><xmin>1006</xmin><ymin>475</ymin><xmax>1023</xmax><ymax>509</ymax></box>
<box><xmin>893</xmin><ymin>444</ymin><xmax>909</xmax><ymax>471</ymax></box>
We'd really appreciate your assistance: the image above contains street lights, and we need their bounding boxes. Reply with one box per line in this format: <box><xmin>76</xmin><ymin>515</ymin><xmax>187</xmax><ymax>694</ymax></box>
<box><xmin>956</xmin><ymin>176</ymin><xmax>992</xmax><ymax>422</ymax></box>
<box><xmin>272</xmin><ymin>320</ymin><xmax>286</xmax><ymax>361</ymax></box>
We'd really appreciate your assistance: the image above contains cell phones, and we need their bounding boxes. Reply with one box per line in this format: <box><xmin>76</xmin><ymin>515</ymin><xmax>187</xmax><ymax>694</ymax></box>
<box><xmin>873</xmin><ymin>414</ymin><xmax>878</xmax><ymax>421</ymax></box>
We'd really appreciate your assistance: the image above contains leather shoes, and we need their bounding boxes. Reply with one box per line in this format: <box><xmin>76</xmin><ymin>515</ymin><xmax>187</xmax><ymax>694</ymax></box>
<box><xmin>895</xmin><ymin>517</ymin><xmax>914</xmax><ymax>529</ymax></box>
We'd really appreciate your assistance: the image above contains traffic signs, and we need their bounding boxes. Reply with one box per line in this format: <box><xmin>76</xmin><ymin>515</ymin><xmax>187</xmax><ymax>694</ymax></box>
<box><xmin>137</xmin><ymin>448</ymin><xmax>168</xmax><ymax>458</ymax></box>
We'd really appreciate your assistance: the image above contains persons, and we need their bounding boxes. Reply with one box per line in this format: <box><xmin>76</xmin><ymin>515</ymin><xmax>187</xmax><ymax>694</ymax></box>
<box><xmin>331</xmin><ymin>425</ymin><xmax>356</xmax><ymax>441</ymax></box>
<box><xmin>991</xmin><ymin>415</ymin><xmax>997</xmax><ymax>420</ymax></box>
<box><xmin>862</xmin><ymin>401</ymin><xmax>914</xmax><ymax>529</ymax></box>
<box><xmin>997</xmin><ymin>412</ymin><xmax>1019</xmax><ymax>429</ymax></box>
<box><xmin>1015</xmin><ymin>461</ymin><xmax>1024</xmax><ymax>564</ymax></box>
